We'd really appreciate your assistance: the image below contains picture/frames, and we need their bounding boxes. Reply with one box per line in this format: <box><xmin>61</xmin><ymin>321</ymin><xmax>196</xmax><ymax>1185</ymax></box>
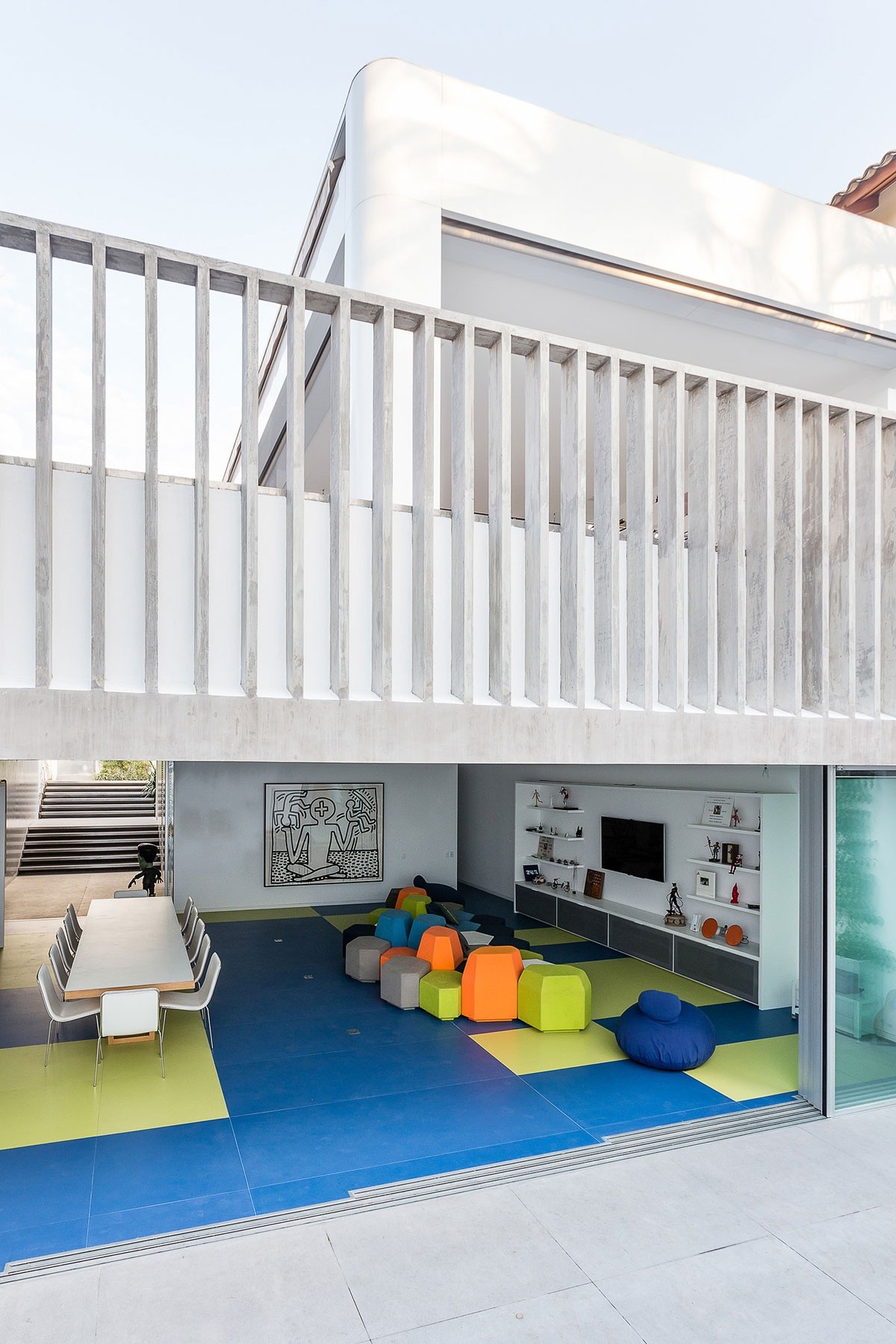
<box><xmin>264</xmin><ymin>783</ymin><xmax>384</xmax><ymax>887</ymax></box>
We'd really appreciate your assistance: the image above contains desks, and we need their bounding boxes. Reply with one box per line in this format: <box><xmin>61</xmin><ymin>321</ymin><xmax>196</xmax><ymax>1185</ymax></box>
<box><xmin>64</xmin><ymin>896</ymin><xmax>195</xmax><ymax>1045</ymax></box>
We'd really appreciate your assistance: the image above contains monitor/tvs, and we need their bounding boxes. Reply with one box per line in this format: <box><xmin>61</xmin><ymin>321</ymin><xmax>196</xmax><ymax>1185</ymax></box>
<box><xmin>601</xmin><ymin>815</ymin><xmax>665</xmax><ymax>883</ymax></box>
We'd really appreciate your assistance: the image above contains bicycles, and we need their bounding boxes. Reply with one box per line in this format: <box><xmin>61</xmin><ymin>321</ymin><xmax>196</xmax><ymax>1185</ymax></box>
<box><xmin>551</xmin><ymin>878</ymin><xmax>570</xmax><ymax>892</ymax></box>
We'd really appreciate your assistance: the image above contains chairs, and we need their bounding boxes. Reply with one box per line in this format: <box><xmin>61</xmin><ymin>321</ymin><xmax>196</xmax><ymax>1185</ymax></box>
<box><xmin>93</xmin><ymin>988</ymin><xmax>166</xmax><ymax>1086</ymax></box>
<box><xmin>615</xmin><ymin>989</ymin><xmax>716</xmax><ymax>1070</ymax></box>
<box><xmin>49</xmin><ymin>944</ymin><xmax>69</xmax><ymax>1034</ymax></box>
<box><xmin>187</xmin><ymin>918</ymin><xmax>205</xmax><ymax>965</ymax></box>
<box><xmin>191</xmin><ymin>933</ymin><xmax>211</xmax><ymax>1020</ymax></box>
<box><xmin>159</xmin><ymin>952</ymin><xmax>221</xmax><ymax>1051</ymax></box>
<box><xmin>63</xmin><ymin>913</ymin><xmax>79</xmax><ymax>957</ymax></box>
<box><xmin>413</xmin><ymin>874</ymin><xmax>465</xmax><ymax>906</ymax></box>
<box><xmin>36</xmin><ymin>963</ymin><xmax>104</xmax><ymax>1066</ymax></box>
<box><xmin>55</xmin><ymin>928</ymin><xmax>74</xmax><ymax>974</ymax></box>
<box><xmin>67</xmin><ymin>903</ymin><xmax>84</xmax><ymax>941</ymax></box>
<box><xmin>114</xmin><ymin>889</ymin><xmax>148</xmax><ymax>898</ymax></box>
<box><xmin>183</xmin><ymin>906</ymin><xmax>199</xmax><ymax>948</ymax></box>
<box><xmin>179</xmin><ymin>896</ymin><xmax>194</xmax><ymax>935</ymax></box>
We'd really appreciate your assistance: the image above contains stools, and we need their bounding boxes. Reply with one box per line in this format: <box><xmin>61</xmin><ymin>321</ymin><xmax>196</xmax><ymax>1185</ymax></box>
<box><xmin>340</xmin><ymin>886</ymin><xmax>592</xmax><ymax>1034</ymax></box>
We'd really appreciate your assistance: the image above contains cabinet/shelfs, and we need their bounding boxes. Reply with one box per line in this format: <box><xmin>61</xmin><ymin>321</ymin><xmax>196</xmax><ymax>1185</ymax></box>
<box><xmin>792</xmin><ymin>954</ymin><xmax>883</xmax><ymax>1039</ymax></box>
<box><xmin>515</xmin><ymin>880</ymin><xmax>759</xmax><ymax>1006</ymax></box>
<box><xmin>687</xmin><ymin>823</ymin><xmax>760</xmax><ymax>915</ymax></box>
<box><xmin>525</xmin><ymin>806</ymin><xmax>584</xmax><ymax>869</ymax></box>
